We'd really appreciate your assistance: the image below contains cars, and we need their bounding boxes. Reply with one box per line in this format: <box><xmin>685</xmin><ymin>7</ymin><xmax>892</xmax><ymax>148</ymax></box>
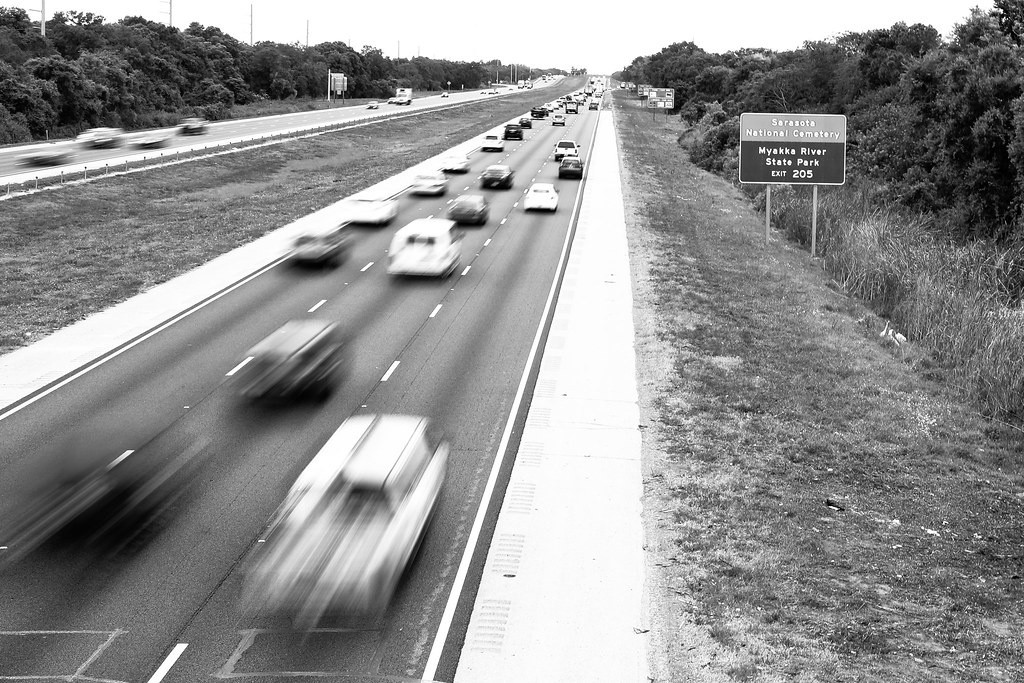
<box><xmin>175</xmin><ymin>117</ymin><xmax>210</xmax><ymax>136</ymax></box>
<box><xmin>365</xmin><ymin>100</ymin><xmax>379</xmax><ymax>109</ymax></box>
<box><xmin>341</xmin><ymin>194</ymin><xmax>401</xmax><ymax>225</ymax></box>
<box><xmin>408</xmin><ymin>169</ymin><xmax>450</xmax><ymax>196</ymax></box>
<box><xmin>480</xmin><ymin>165</ymin><xmax>517</xmax><ymax>189</ymax></box>
<box><xmin>481</xmin><ymin>133</ymin><xmax>506</xmax><ymax>152</ymax></box>
<box><xmin>286</xmin><ymin>229</ymin><xmax>354</xmax><ymax>269</ymax></box>
<box><xmin>16</xmin><ymin>125</ymin><xmax>172</xmax><ymax>165</ymax></box>
<box><xmin>440</xmin><ymin>73</ymin><xmax>605</xmax><ymax>129</ymax></box>
<box><xmin>522</xmin><ymin>182</ymin><xmax>560</xmax><ymax>212</ymax></box>
<box><xmin>553</xmin><ymin>140</ymin><xmax>580</xmax><ymax>161</ymax></box>
<box><xmin>387</xmin><ymin>98</ymin><xmax>396</xmax><ymax>103</ymax></box>
<box><xmin>558</xmin><ymin>155</ymin><xmax>584</xmax><ymax>178</ymax></box>
<box><xmin>231</xmin><ymin>318</ymin><xmax>354</xmax><ymax>408</ymax></box>
<box><xmin>503</xmin><ymin>123</ymin><xmax>524</xmax><ymax>140</ymax></box>
<box><xmin>386</xmin><ymin>217</ymin><xmax>468</xmax><ymax>277</ymax></box>
<box><xmin>447</xmin><ymin>195</ymin><xmax>493</xmax><ymax>225</ymax></box>
<box><xmin>441</xmin><ymin>152</ymin><xmax>472</xmax><ymax>172</ymax></box>
<box><xmin>234</xmin><ymin>412</ymin><xmax>454</xmax><ymax>617</ymax></box>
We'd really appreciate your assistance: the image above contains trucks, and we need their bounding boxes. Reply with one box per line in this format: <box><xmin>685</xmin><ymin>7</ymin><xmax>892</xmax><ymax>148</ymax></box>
<box><xmin>395</xmin><ymin>87</ymin><xmax>412</xmax><ymax>106</ymax></box>
